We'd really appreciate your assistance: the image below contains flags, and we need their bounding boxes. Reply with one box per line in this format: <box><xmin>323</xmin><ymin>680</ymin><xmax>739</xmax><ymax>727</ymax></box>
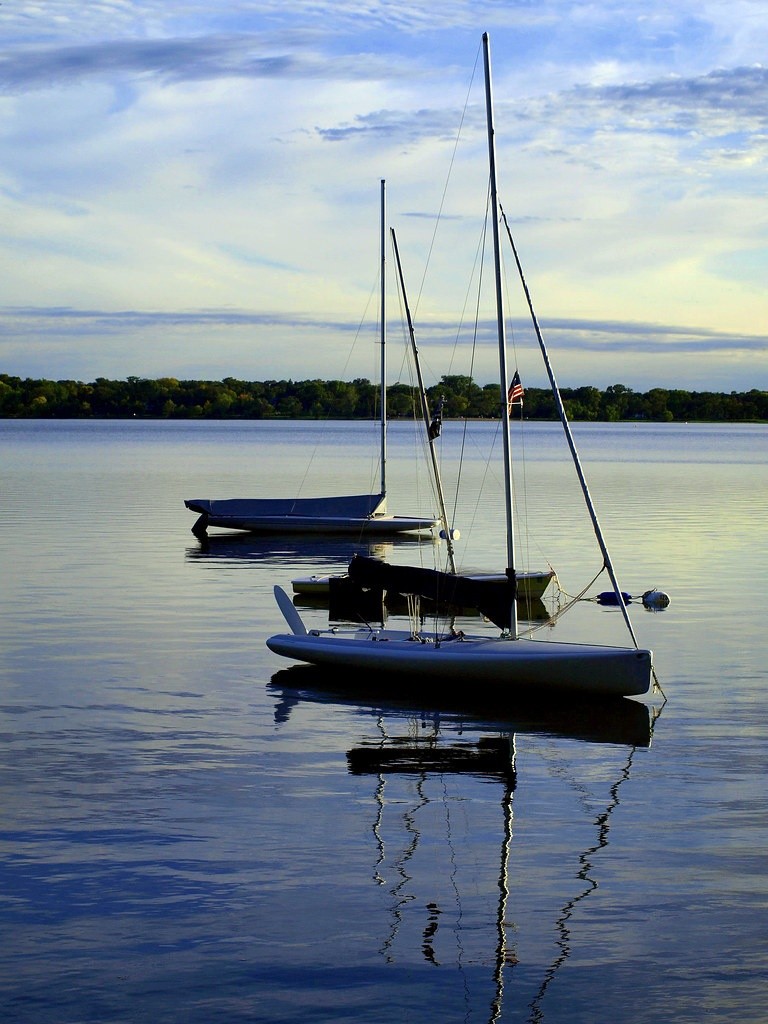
<box><xmin>429</xmin><ymin>394</ymin><xmax>443</xmax><ymax>441</ymax></box>
<box><xmin>508</xmin><ymin>370</ymin><xmax>524</xmax><ymax>417</ymax></box>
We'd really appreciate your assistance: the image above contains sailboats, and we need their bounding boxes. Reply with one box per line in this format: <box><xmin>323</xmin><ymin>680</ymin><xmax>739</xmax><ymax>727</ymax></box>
<box><xmin>185</xmin><ymin>177</ymin><xmax>444</xmax><ymax>539</ymax></box>
<box><xmin>291</xmin><ymin>228</ymin><xmax>558</xmax><ymax>619</ymax></box>
<box><xmin>265</xmin><ymin>30</ymin><xmax>660</xmax><ymax>705</ymax></box>
